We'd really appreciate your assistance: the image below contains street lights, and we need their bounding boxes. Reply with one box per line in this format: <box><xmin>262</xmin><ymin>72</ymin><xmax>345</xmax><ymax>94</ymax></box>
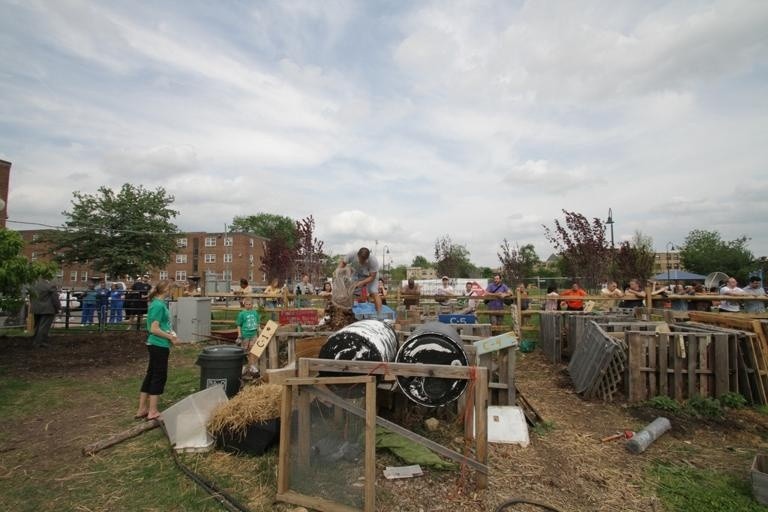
<box><xmin>223</xmin><ymin>221</ymin><xmax>239</xmax><ymax>308</ymax></box>
<box><xmin>372</xmin><ymin>238</ymin><xmax>395</xmax><ymax>294</ymax></box>
<box><xmin>666</xmin><ymin>240</ymin><xmax>674</xmax><ymax>292</ymax></box>
<box><xmin>604</xmin><ymin>206</ymin><xmax>616</xmax><ymax>281</ymax></box>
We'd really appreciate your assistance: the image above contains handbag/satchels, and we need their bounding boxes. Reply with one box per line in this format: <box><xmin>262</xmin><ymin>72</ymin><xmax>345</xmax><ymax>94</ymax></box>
<box><xmin>484</xmin><ymin>299</ymin><xmax>490</xmax><ymax>304</ymax></box>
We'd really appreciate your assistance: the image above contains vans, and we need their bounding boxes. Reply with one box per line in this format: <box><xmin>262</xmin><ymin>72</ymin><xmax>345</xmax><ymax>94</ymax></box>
<box><xmin>95</xmin><ymin>281</ymin><xmax>127</xmax><ymax>305</ymax></box>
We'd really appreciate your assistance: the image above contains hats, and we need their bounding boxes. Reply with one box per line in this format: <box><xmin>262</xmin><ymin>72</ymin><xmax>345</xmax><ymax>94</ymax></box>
<box><xmin>442</xmin><ymin>276</ymin><xmax>448</xmax><ymax>281</ymax></box>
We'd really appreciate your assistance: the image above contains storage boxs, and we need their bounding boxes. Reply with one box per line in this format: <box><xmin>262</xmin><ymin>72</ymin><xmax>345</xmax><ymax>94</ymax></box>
<box><xmin>160</xmin><ymin>384</ymin><xmax>234</xmax><ymax>454</ymax></box>
<box><xmin>750</xmin><ymin>453</ymin><xmax>768</xmax><ymax>509</ymax></box>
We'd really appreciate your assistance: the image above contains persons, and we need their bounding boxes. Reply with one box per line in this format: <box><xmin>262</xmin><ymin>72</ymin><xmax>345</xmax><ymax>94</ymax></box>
<box><xmin>235</xmin><ymin>278</ymin><xmax>252</xmax><ymax>308</ymax></box>
<box><xmin>236</xmin><ymin>298</ymin><xmax>260</xmax><ymax>372</ymax></box>
<box><xmin>126</xmin><ymin>275</ymin><xmax>153</xmax><ymax>330</ymax></box>
<box><xmin>135</xmin><ymin>280</ymin><xmax>177</xmax><ymax>419</ymax></box>
<box><xmin>516</xmin><ymin>285</ymin><xmax>531</xmax><ymax>325</ymax></box>
<box><xmin>651</xmin><ymin>277</ymin><xmax>768</xmax><ymax>313</ymax></box>
<box><xmin>601</xmin><ymin>279</ymin><xmax>647</xmax><ymax>314</ymax></box>
<box><xmin>485</xmin><ymin>275</ymin><xmax>513</xmax><ymax>325</ymax></box>
<box><xmin>463</xmin><ymin>282</ymin><xmax>480</xmax><ymax>315</ymax></box>
<box><xmin>29</xmin><ymin>272</ymin><xmax>61</xmax><ymax>351</ymax></box>
<box><xmin>292</xmin><ymin>274</ymin><xmax>317</xmax><ymax>295</ymax></box>
<box><xmin>337</xmin><ymin>247</ymin><xmax>382</xmax><ymax>320</ymax></box>
<box><xmin>402</xmin><ymin>278</ymin><xmax>420</xmax><ymax>309</ymax></box>
<box><xmin>544</xmin><ymin>283</ymin><xmax>587</xmax><ymax>311</ymax></box>
<box><xmin>357</xmin><ymin>278</ymin><xmax>387</xmax><ymax>305</ymax></box>
<box><xmin>318</xmin><ymin>282</ymin><xmax>333</xmax><ymax>295</ymax></box>
<box><xmin>435</xmin><ymin>276</ymin><xmax>455</xmax><ymax>315</ymax></box>
<box><xmin>263</xmin><ymin>279</ymin><xmax>281</xmax><ymax>308</ymax></box>
<box><xmin>80</xmin><ymin>281</ymin><xmax>123</xmax><ymax>326</ymax></box>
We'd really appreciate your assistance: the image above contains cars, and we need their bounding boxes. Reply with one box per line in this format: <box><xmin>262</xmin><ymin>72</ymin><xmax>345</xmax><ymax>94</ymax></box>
<box><xmin>197</xmin><ymin>286</ymin><xmax>235</xmax><ymax>300</ymax></box>
<box><xmin>54</xmin><ymin>292</ymin><xmax>80</xmax><ymax>313</ymax></box>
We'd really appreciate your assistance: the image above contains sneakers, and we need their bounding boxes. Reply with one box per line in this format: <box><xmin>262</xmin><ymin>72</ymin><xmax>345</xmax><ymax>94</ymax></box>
<box><xmin>242</xmin><ymin>364</ymin><xmax>248</xmax><ymax>375</ymax></box>
<box><xmin>249</xmin><ymin>366</ymin><xmax>259</xmax><ymax>373</ymax></box>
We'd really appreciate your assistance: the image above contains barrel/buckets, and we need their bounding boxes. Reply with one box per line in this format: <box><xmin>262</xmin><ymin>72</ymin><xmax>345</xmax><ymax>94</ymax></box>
<box><xmin>319</xmin><ymin>318</ymin><xmax>398</xmax><ymax>399</ymax></box>
<box><xmin>395</xmin><ymin>320</ymin><xmax>471</xmax><ymax>408</ymax></box>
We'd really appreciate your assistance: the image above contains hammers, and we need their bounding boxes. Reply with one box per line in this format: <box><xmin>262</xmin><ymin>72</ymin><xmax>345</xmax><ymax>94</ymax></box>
<box><xmin>601</xmin><ymin>431</ymin><xmax>626</xmax><ymax>442</ymax></box>
